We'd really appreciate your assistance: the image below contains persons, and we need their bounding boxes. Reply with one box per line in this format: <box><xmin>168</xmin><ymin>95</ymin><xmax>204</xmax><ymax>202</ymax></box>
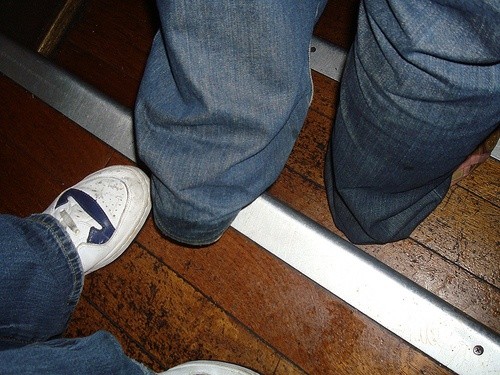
<box><xmin>132</xmin><ymin>0</ymin><xmax>500</xmax><ymax>247</ymax></box>
<box><xmin>0</xmin><ymin>165</ymin><xmax>260</xmax><ymax>375</ymax></box>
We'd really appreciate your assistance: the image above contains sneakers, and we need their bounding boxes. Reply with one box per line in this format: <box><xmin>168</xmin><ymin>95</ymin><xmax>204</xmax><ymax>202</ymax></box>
<box><xmin>41</xmin><ymin>164</ymin><xmax>152</xmax><ymax>277</ymax></box>
<box><xmin>160</xmin><ymin>359</ymin><xmax>259</xmax><ymax>375</ymax></box>
